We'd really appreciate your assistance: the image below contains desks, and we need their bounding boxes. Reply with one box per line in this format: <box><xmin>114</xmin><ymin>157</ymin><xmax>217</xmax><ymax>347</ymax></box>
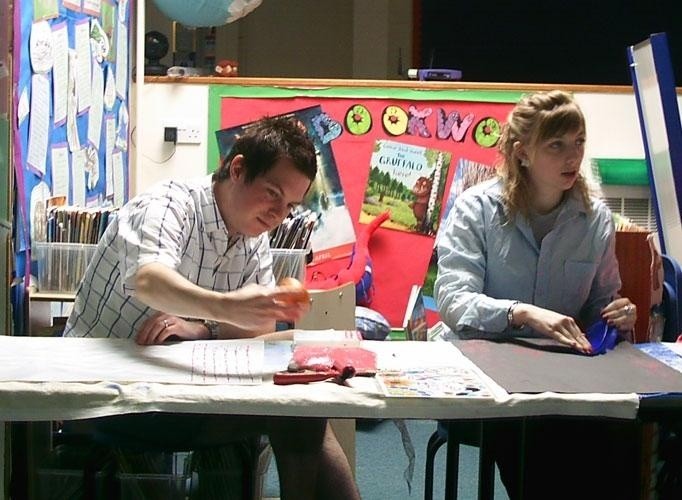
<box><xmin>1</xmin><ymin>332</ymin><xmax>681</xmax><ymax>499</ymax></box>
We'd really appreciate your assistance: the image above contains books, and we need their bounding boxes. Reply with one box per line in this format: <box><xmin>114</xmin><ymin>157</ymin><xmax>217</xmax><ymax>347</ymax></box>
<box><xmin>35</xmin><ymin>203</ymin><xmax>121</xmax><ymax>292</ymax></box>
<box><xmin>402</xmin><ymin>284</ymin><xmax>427</xmax><ymax>341</ymax></box>
<box><xmin>267</xmin><ymin>206</ymin><xmax>317</xmax><ymax>287</ymax></box>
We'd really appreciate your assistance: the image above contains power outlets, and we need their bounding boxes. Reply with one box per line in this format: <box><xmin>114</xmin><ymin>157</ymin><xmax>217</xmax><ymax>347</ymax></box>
<box><xmin>176</xmin><ymin>126</ymin><xmax>204</xmax><ymax>144</ymax></box>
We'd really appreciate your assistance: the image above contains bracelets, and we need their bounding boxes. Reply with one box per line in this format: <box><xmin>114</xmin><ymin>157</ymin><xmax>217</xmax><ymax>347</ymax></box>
<box><xmin>508</xmin><ymin>300</ymin><xmax>528</xmax><ymax>330</ymax></box>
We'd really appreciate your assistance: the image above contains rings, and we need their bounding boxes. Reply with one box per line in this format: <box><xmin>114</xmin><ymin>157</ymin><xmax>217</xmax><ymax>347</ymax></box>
<box><xmin>625</xmin><ymin>305</ymin><xmax>630</xmax><ymax>314</ymax></box>
<box><xmin>163</xmin><ymin>318</ymin><xmax>170</xmax><ymax>328</ymax></box>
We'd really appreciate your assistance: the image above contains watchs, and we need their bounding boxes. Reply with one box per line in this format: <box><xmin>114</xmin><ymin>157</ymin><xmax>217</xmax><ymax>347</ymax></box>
<box><xmin>204</xmin><ymin>320</ymin><xmax>220</xmax><ymax>340</ymax></box>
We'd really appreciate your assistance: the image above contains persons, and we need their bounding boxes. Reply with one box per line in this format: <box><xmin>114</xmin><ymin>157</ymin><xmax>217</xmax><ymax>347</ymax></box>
<box><xmin>433</xmin><ymin>90</ymin><xmax>644</xmax><ymax>499</ymax></box>
<box><xmin>61</xmin><ymin>117</ymin><xmax>360</xmax><ymax>499</ymax></box>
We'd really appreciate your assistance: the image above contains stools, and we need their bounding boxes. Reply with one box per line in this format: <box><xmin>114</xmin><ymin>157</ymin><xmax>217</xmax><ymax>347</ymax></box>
<box><xmin>422</xmin><ymin>421</ymin><xmax>479</xmax><ymax>499</ymax></box>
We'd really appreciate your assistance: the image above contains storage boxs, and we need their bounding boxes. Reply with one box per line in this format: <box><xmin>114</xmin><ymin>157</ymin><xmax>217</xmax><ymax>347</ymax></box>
<box><xmin>33</xmin><ymin>241</ymin><xmax>97</xmax><ymax>294</ymax></box>
<box><xmin>32</xmin><ymin>446</ymin><xmax>271</xmax><ymax>498</ymax></box>
<box><xmin>270</xmin><ymin>247</ymin><xmax>312</xmax><ymax>291</ymax></box>
